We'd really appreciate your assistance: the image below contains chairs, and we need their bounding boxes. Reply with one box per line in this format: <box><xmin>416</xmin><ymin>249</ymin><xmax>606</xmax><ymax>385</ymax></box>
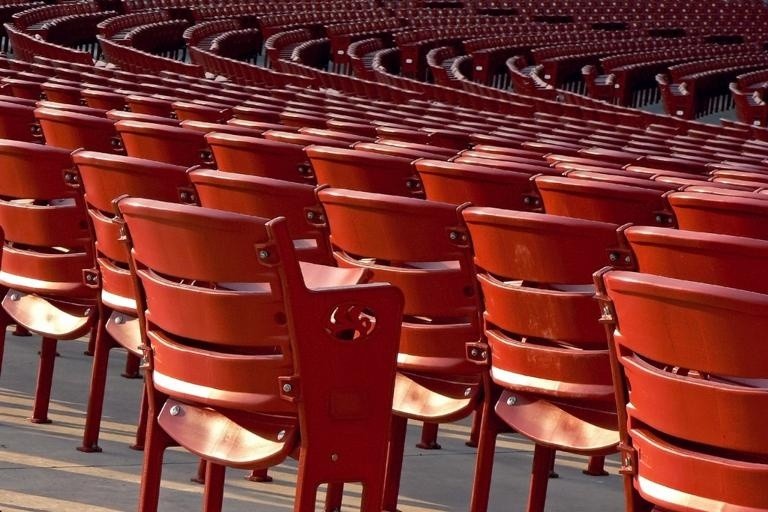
<box><xmin>0</xmin><ymin>1</ymin><xmax>767</xmax><ymax>512</ymax></box>
